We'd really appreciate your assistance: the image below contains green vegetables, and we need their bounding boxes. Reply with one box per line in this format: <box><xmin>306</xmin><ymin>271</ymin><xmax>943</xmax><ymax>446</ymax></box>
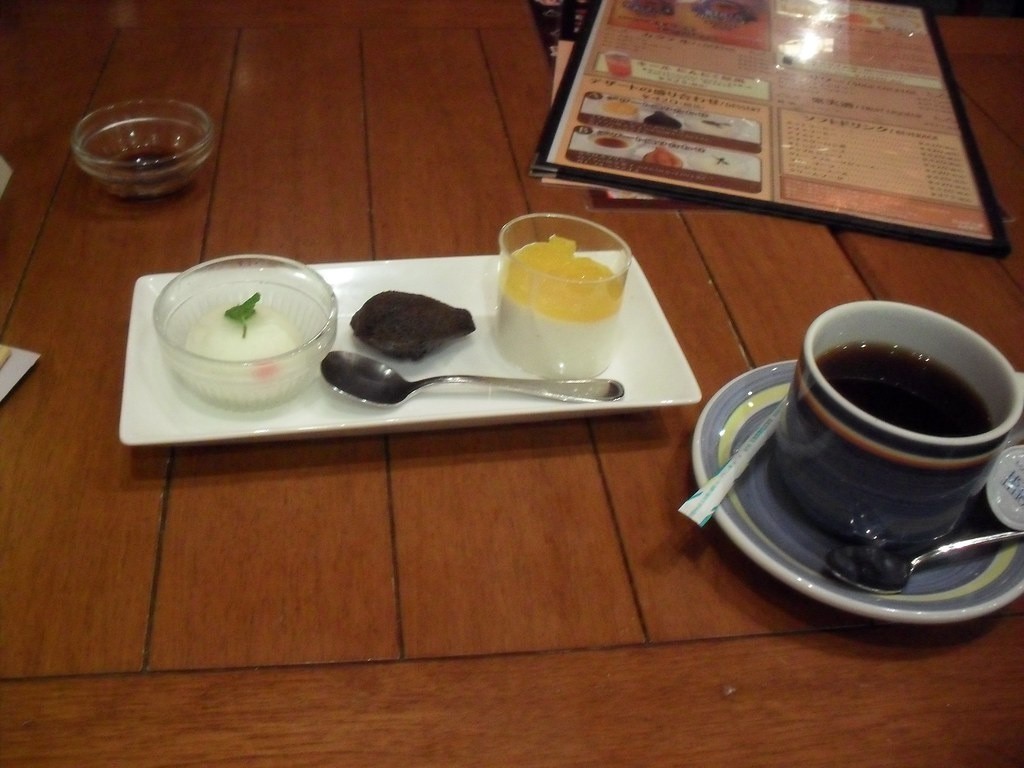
<box><xmin>225</xmin><ymin>292</ymin><xmax>261</xmax><ymax>339</ymax></box>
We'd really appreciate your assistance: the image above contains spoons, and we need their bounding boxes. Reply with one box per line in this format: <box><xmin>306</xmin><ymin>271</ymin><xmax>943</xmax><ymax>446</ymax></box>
<box><xmin>321</xmin><ymin>349</ymin><xmax>626</xmax><ymax>410</ymax></box>
<box><xmin>821</xmin><ymin>528</ymin><xmax>1024</xmax><ymax>595</ymax></box>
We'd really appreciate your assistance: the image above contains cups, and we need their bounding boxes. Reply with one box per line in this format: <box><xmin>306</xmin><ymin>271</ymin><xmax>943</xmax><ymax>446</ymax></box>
<box><xmin>492</xmin><ymin>211</ymin><xmax>632</xmax><ymax>382</ymax></box>
<box><xmin>774</xmin><ymin>300</ymin><xmax>1023</xmax><ymax>549</ymax></box>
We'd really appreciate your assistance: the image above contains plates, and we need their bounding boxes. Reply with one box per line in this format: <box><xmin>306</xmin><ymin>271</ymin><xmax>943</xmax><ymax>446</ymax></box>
<box><xmin>117</xmin><ymin>250</ymin><xmax>702</xmax><ymax>447</ymax></box>
<box><xmin>691</xmin><ymin>359</ymin><xmax>1024</xmax><ymax>625</ymax></box>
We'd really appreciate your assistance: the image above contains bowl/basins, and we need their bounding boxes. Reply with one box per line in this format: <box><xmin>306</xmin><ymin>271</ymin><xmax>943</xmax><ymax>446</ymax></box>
<box><xmin>153</xmin><ymin>253</ymin><xmax>339</xmax><ymax>414</ymax></box>
<box><xmin>70</xmin><ymin>97</ymin><xmax>220</xmax><ymax>203</ymax></box>
<box><xmin>588</xmin><ymin>129</ymin><xmax>637</xmax><ymax>156</ymax></box>
